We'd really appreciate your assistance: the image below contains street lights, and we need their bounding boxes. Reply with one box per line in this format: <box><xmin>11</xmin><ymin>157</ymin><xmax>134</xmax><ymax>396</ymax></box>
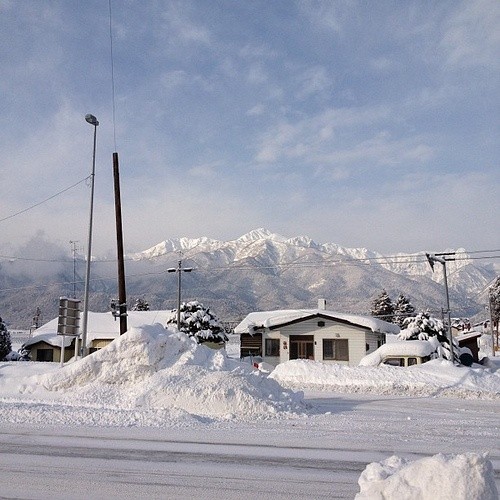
<box><xmin>82</xmin><ymin>113</ymin><xmax>100</xmax><ymax>360</ymax></box>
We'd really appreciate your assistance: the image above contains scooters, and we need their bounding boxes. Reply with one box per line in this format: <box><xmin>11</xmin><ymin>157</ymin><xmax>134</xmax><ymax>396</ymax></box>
<box><xmin>20</xmin><ymin>349</ymin><xmax>32</xmax><ymax>361</ymax></box>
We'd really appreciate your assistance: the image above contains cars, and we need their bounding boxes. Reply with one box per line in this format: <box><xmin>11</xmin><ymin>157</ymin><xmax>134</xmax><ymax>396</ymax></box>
<box><xmin>357</xmin><ymin>338</ymin><xmax>431</xmax><ymax>367</ymax></box>
